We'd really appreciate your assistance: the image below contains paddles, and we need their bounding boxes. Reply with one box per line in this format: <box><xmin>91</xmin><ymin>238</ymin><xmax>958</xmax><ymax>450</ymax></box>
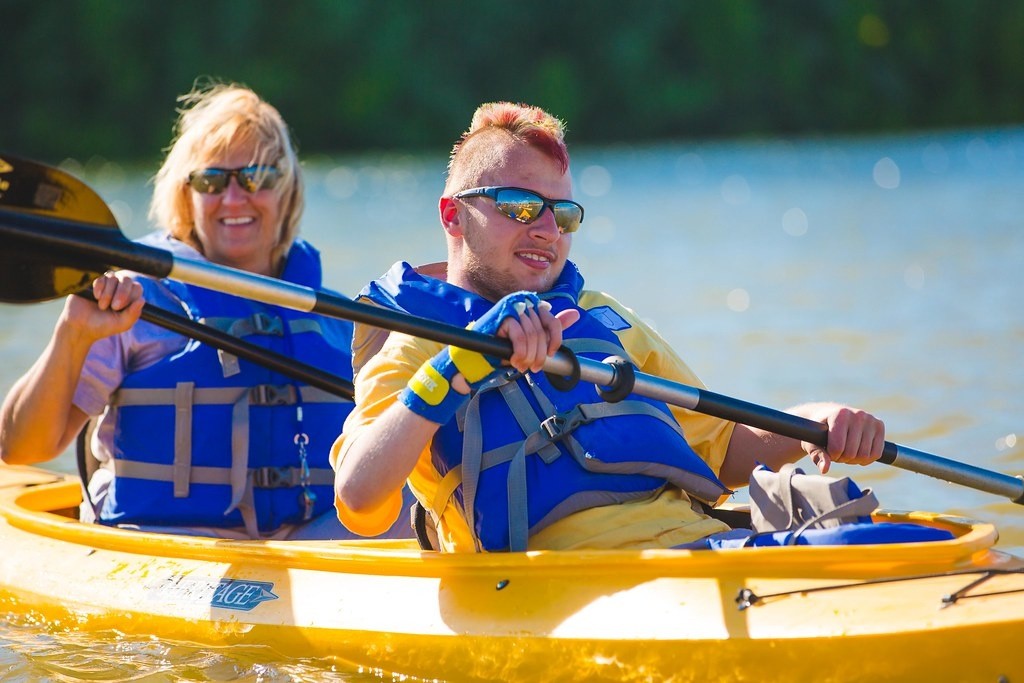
<box><xmin>0</xmin><ymin>154</ymin><xmax>1023</xmax><ymax>507</ymax></box>
<box><xmin>73</xmin><ymin>278</ymin><xmax>354</xmax><ymax>402</ymax></box>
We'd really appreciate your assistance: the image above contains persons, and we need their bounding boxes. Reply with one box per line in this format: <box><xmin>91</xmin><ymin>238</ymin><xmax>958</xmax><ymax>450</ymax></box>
<box><xmin>0</xmin><ymin>85</ymin><xmax>418</xmax><ymax>541</ymax></box>
<box><xmin>329</xmin><ymin>102</ymin><xmax>957</xmax><ymax>553</ymax></box>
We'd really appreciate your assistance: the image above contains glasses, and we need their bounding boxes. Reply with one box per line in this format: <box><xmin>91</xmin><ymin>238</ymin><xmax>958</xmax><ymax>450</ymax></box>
<box><xmin>186</xmin><ymin>162</ymin><xmax>285</xmax><ymax>195</ymax></box>
<box><xmin>454</xmin><ymin>184</ymin><xmax>584</xmax><ymax>234</ymax></box>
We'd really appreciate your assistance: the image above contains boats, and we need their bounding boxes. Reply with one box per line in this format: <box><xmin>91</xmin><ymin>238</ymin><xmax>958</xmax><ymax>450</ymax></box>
<box><xmin>0</xmin><ymin>458</ymin><xmax>1024</xmax><ymax>683</ymax></box>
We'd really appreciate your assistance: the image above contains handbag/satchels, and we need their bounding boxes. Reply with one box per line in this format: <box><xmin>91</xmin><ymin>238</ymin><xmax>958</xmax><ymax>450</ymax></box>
<box><xmin>748</xmin><ymin>464</ymin><xmax>878</xmax><ymax>544</ymax></box>
